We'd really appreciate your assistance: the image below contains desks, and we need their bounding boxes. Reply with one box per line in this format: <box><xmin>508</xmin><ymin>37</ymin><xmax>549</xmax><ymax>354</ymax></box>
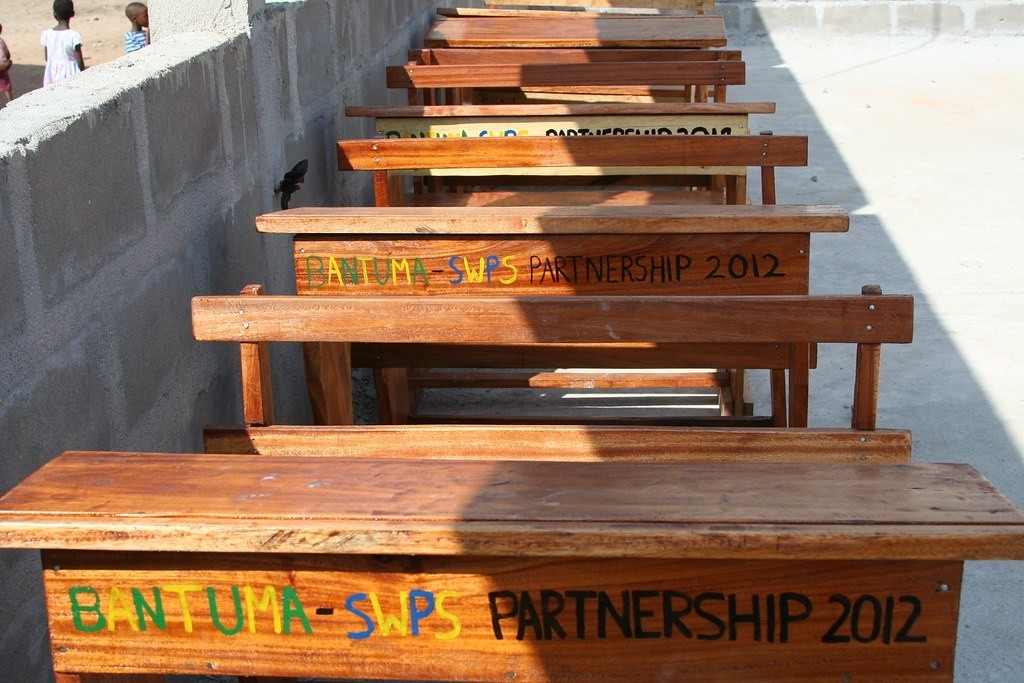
<box><xmin>0</xmin><ymin>448</ymin><xmax>1024</xmax><ymax>683</ymax></box>
<box><xmin>420</xmin><ymin>14</ymin><xmax>725</xmax><ymax>105</ymax></box>
<box><xmin>260</xmin><ymin>206</ymin><xmax>850</xmax><ymax>426</ymax></box>
<box><xmin>343</xmin><ymin>103</ymin><xmax>777</xmax><ymax>207</ymax></box>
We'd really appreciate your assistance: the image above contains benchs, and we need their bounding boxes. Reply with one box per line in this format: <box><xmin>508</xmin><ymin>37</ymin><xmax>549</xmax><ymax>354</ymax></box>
<box><xmin>185</xmin><ymin>285</ymin><xmax>916</xmax><ymax>463</ymax></box>
<box><xmin>336</xmin><ymin>132</ymin><xmax>808</xmax><ymax>207</ymax></box>
<box><xmin>386</xmin><ymin>61</ymin><xmax>746</xmax><ymax>193</ymax></box>
<box><xmin>437</xmin><ymin>1</ymin><xmax>717</xmax><ymax>100</ymax></box>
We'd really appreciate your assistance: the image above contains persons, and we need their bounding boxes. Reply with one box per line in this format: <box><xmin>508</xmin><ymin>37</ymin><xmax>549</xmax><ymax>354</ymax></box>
<box><xmin>40</xmin><ymin>0</ymin><xmax>85</xmax><ymax>87</ymax></box>
<box><xmin>124</xmin><ymin>2</ymin><xmax>150</xmax><ymax>55</ymax></box>
<box><xmin>0</xmin><ymin>24</ymin><xmax>13</xmax><ymax>102</ymax></box>
